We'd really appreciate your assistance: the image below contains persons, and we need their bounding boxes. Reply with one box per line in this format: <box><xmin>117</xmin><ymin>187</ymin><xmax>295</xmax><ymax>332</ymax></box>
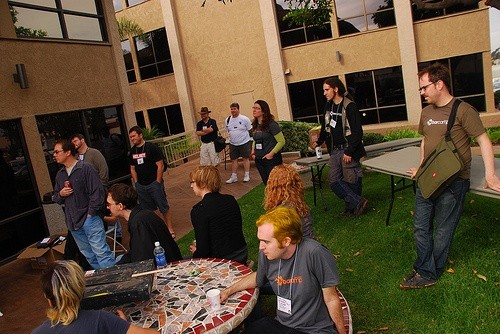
<box><xmin>250</xmin><ymin>100</ymin><xmax>286</xmax><ymax>187</ymax></box>
<box><xmin>398</xmin><ymin>63</ymin><xmax>500</xmax><ymax>289</ymax></box>
<box><xmin>31</xmin><ymin>260</ymin><xmax>161</xmax><ymax>334</ymax></box>
<box><xmin>207</xmin><ymin>207</ymin><xmax>348</xmax><ymax>334</ymax></box>
<box><xmin>225</xmin><ymin>103</ymin><xmax>253</xmax><ymax>184</ymax></box>
<box><xmin>264</xmin><ymin>163</ymin><xmax>318</xmax><ymax>241</ymax></box>
<box><xmin>189</xmin><ymin>166</ymin><xmax>254</xmax><ymax>270</ymax></box>
<box><xmin>196</xmin><ymin>107</ymin><xmax>220</xmax><ymax>167</ymax></box>
<box><xmin>312</xmin><ymin>77</ymin><xmax>368</xmax><ymax>218</ymax></box>
<box><xmin>52</xmin><ymin>138</ymin><xmax>116</xmax><ymax>269</ymax></box>
<box><xmin>105</xmin><ymin>182</ymin><xmax>183</xmax><ymax>265</ymax></box>
<box><xmin>71</xmin><ymin>133</ymin><xmax>124</xmax><ymax>250</ymax></box>
<box><xmin>129</xmin><ymin>126</ymin><xmax>176</xmax><ymax>239</ymax></box>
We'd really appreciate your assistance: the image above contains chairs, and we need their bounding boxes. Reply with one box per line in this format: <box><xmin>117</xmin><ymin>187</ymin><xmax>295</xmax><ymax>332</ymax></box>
<box><xmin>103</xmin><ymin>222</ymin><xmax>128</xmax><ymax>257</ymax></box>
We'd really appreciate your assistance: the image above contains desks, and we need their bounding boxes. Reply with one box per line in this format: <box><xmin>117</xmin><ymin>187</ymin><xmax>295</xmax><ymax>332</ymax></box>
<box><xmin>293</xmin><ymin>138</ymin><xmax>500</xmax><ymax>226</ymax></box>
<box><xmin>117</xmin><ymin>258</ymin><xmax>259</xmax><ymax>334</ymax></box>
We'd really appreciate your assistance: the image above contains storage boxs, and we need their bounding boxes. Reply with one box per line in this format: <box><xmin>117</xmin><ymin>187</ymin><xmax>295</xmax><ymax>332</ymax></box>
<box><xmin>16</xmin><ymin>232</ymin><xmax>68</xmax><ymax>270</ymax></box>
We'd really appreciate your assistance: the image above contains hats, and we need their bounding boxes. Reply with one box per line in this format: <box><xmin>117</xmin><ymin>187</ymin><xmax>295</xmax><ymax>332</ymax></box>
<box><xmin>198</xmin><ymin>106</ymin><xmax>212</xmax><ymax>114</ymax></box>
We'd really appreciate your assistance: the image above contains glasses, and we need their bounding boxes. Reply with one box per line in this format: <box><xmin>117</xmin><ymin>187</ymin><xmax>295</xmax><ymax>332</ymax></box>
<box><xmin>252</xmin><ymin>107</ymin><xmax>262</xmax><ymax>110</ymax></box>
<box><xmin>418</xmin><ymin>79</ymin><xmax>438</xmax><ymax>92</ymax></box>
<box><xmin>53</xmin><ymin>149</ymin><xmax>64</xmax><ymax>155</ymax></box>
<box><xmin>106</xmin><ymin>202</ymin><xmax>118</xmax><ymax>208</ymax></box>
<box><xmin>190</xmin><ymin>181</ymin><xmax>198</xmax><ymax>184</ymax></box>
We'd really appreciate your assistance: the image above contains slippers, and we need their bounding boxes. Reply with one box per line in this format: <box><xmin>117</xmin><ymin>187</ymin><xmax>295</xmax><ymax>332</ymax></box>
<box><xmin>171</xmin><ymin>232</ymin><xmax>176</xmax><ymax>239</ymax></box>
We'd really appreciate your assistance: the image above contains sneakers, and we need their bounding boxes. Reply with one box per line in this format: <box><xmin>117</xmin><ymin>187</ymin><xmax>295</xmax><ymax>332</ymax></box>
<box><xmin>340</xmin><ymin>207</ymin><xmax>355</xmax><ymax>216</ymax></box>
<box><xmin>400</xmin><ymin>271</ymin><xmax>436</xmax><ymax>288</ymax></box>
<box><xmin>226</xmin><ymin>176</ymin><xmax>238</xmax><ymax>183</ymax></box>
<box><xmin>404</xmin><ymin>268</ymin><xmax>418</xmax><ymax>283</ymax></box>
<box><xmin>354</xmin><ymin>197</ymin><xmax>368</xmax><ymax>217</ymax></box>
<box><xmin>243</xmin><ymin>176</ymin><xmax>250</xmax><ymax>182</ymax></box>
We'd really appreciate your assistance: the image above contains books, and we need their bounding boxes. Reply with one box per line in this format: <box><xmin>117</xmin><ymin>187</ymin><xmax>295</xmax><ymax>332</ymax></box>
<box><xmin>36</xmin><ymin>234</ymin><xmax>66</xmax><ymax>248</ymax></box>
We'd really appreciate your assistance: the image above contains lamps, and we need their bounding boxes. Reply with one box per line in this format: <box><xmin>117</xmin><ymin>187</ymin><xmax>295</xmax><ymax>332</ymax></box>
<box><xmin>13</xmin><ymin>63</ymin><xmax>30</xmax><ymax>89</ymax></box>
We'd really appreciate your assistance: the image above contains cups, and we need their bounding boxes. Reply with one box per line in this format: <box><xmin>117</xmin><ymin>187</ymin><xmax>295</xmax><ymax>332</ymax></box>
<box><xmin>206</xmin><ymin>289</ymin><xmax>221</xmax><ymax>312</ymax></box>
<box><xmin>226</xmin><ymin>138</ymin><xmax>229</xmax><ymax>143</ymax></box>
<box><xmin>315</xmin><ymin>145</ymin><xmax>322</xmax><ymax>159</ymax></box>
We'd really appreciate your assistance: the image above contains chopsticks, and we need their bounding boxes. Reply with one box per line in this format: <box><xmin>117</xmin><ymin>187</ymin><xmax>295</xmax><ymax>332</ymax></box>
<box><xmin>131</xmin><ymin>269</ymin><xmax>167</xmax><ymax>278</ymax></box>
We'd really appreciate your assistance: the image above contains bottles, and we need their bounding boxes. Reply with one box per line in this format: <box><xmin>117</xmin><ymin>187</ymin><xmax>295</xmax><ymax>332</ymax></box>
<box><xmin>154</xmin><ymin>241</ymin><xmax>168</xmax><ymax>270</ymax></box>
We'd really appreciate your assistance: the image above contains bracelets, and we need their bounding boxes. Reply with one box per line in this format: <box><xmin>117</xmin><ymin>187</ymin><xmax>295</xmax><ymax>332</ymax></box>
<box><xmin>250</xmin><ymin>153</ymin><xmax>255</xmax><ymax>158</ymax></box>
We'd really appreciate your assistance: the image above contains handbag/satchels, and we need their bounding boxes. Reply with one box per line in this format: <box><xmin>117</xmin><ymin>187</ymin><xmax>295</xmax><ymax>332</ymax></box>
<box><xmin>415</xmin><ymin>140</ymin><xmax>464</xmax><ymax>202</ymax></box>
<box><xmin>213</xmin><ymin>135</ymin><xmax>227</xmax><ymax>153</ymax></box>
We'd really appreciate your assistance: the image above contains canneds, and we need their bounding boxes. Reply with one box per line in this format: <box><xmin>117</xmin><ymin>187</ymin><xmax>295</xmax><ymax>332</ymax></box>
<box><xmin>64</xmin><ymin>181</ymin><xmax>72</xmax><ymax>189</ymax></box>
<box><xmin>315</xmin><ymin>146</ymin><xmax>322</xmax><ymax>159</ymax></box>
